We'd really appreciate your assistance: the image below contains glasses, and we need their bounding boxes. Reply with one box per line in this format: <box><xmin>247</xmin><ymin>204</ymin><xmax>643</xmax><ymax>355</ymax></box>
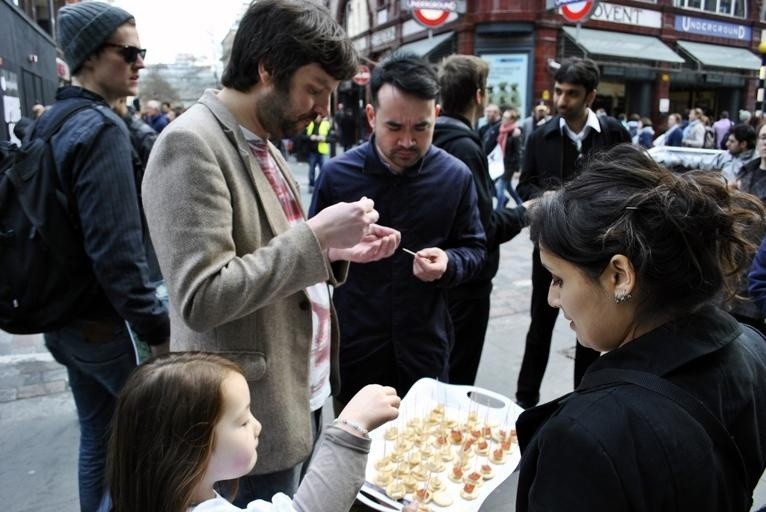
<box><xmin>101</xmin><ymin>40</ymin><xmax>146</xmax><ymax>63</ymax></box>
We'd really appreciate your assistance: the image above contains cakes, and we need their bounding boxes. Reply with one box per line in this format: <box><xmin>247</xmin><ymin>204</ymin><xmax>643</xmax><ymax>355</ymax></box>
<box><xmin>365</xmin><ymin>403</ymin><xmax>520</xmax><ymax>511</ymax></box>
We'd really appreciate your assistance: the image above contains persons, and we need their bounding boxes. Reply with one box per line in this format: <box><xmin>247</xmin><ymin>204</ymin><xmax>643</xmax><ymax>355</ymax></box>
<box><xmin>275</xmin><ymin>98</ymin><xmax>372</xmax><ymax>194</ymax></box>
<box><xmin>30</xmin><ymin>104</ymin><xmax>45</xmax><ymax>117</ymax></box>
<box><xmin>518</xmin><ymin>55</ymin><xmax>634</xmax><ymax>409</ymax></box>
<box><xmin>113</xmin><ymin>94</ymin><xmax>185</xmax><ymax>284</ymax></box>
<box><xmin>307</xmin><ymin>54</ymin><xmax>486</xmax><ymax>419</ymax></box>
<box><xmin>91</xmin><ymin>350</ymin><xmax>400</xmax><ymax>511</ymax></box>
<box><xmin>598</xmin><ymin>99</ymin><xmax>765</xmax><ymax>326</ymax></box>
<box><xmin>0</xmin><ymin>3</ymin><xmax>168</xmax><ymax>511</ymax></box>
<box><xmin>515</xmin><ymin>141</ymin><xmax>766</xmax><ymax>512</ymax></box>
<box><xmin>476</xmin><ymin>103</ymin><xmax>550</xmax><ymax>210</ymax></box>
<box><xmin>141</xmin><ymin>0</ymin><xmax>402</xmax><ymax>507</ymax></box>
<box><xmin>429</xmin><ymin>55</ymin><xmax>547</xmax><ymax>385</ymax></box>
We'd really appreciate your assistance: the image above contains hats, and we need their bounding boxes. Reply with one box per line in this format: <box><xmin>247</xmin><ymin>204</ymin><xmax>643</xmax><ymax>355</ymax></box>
<box><xmin>57</xmin><ymin>0</ymin><xmax>134</xmax><ymax>77</ymax></box>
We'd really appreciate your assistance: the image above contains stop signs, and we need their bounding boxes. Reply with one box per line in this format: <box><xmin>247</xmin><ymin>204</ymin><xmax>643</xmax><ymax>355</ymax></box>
<box><xmin>352</xmin><ymin>64</ymin><xmax>370</xmax><ymax>86</ymax></box>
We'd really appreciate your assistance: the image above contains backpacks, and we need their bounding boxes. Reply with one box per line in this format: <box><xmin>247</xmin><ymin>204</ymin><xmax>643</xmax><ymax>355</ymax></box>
<box><xmin>1</xmin><ymin>99</ymin><xmax>143</xmax><ymax>334</ymax></box>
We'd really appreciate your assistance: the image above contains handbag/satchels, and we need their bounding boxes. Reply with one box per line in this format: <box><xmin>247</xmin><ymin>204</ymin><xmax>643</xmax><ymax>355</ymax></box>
<box><xmin>487</xmin><ymin>143</ymin><xmax>505</xmax><ymax>181</ymax></box>
